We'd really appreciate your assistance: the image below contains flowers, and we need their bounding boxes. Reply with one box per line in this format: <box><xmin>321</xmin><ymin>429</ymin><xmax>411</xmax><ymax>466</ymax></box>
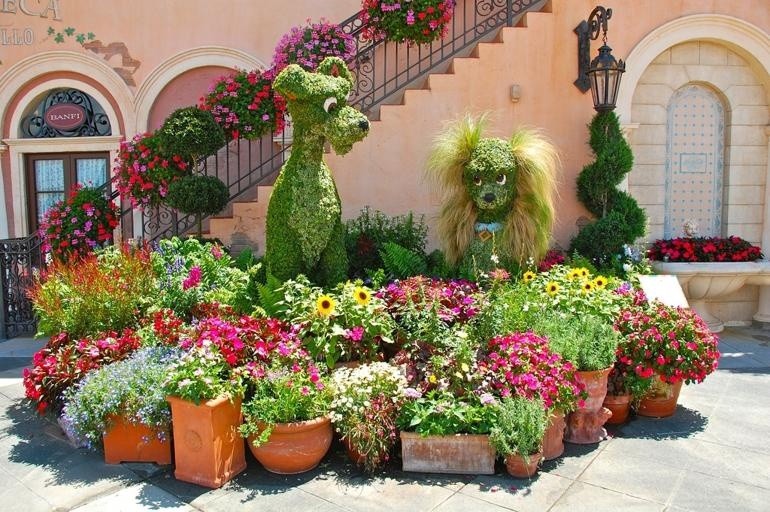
<box><xmin>198</xmin><ymin>65</ymin><xmax>289</xmax><ymax>140</ymax></box>
<box><xmin>357</xmin><ymin>0</ymin><xmax>455</xmax><ymax>49</ymax></box>
<box><xmin>269</xmin><ymin>16</ymin><xmax>357</xmax><ymax>98</ymax></box>
<box><xmin>37</xmin><ymin>181</ymin><xmax>121</xmax><ymax>264</ymax></box>
<box><xmin>645</xmin><ymin>234</ymin><xmax>764</xmax><ymax>261</ymax></box>
<box><xmin>111</xmin><ymin>131</ymin><xmax>191</xmax><ymax>214</ymax></box>
